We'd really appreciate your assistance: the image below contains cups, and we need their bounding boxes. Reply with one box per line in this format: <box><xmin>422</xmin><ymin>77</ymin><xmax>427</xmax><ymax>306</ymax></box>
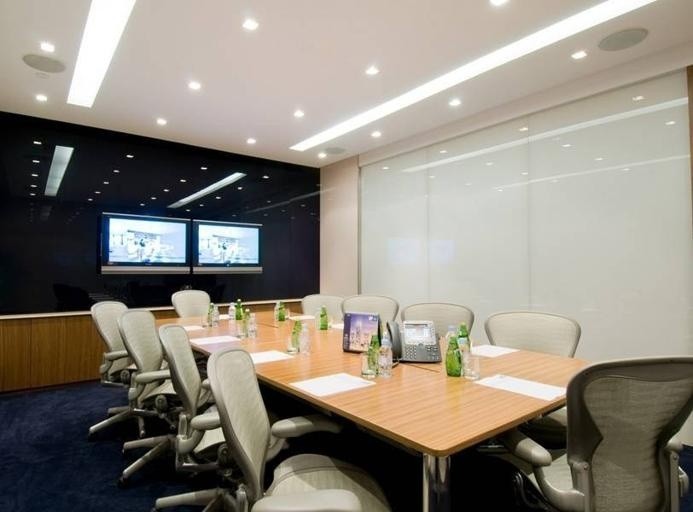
<box><xmin>201</xmin><ymin>311</ymin><xmax>213</xmax><ymax>326</ymax></box>
<box><xmin>283</xmin><ymin>305</ymin><xmax>290</xmax><ymax>318</ymax></box>
<box><xmin>464</xmin><ymin>354</ymin><xmax>481</xmax><ymax>379</ymax></box>
<box><xmin>360</xmin><ymin>353</ymin><xmax>377</xmax><ymax>378</ymax></box>
<box><xmin>235</xmin><ymin>320</ymin><xmax>250</xmax><ymax>339</ymax></box>
<box><xmin>286</xmin><ymin>333</ymin><xmax>298</xmax><ymax>353</ymax></box>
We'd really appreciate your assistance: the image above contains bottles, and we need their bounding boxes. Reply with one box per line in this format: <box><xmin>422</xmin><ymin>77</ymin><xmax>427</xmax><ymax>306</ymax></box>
<box><xmin>279</xmin><ymin>302</ymin><xmax>285</xmax><ymax>321</ymax></box>
<box><xmin>445</xmin><ymin>325</ymin><xmax>457</xmax><ymax>361</ymax></box>
<box><xmin>243</xmin><ymin>309</ymin><xmax>250</xmax><ymax>338</ymax></box>
<box><xmin>458</xmin><ymin>322</ymin><xmax>470</xmax><ymax>353</ymax></box>
<box><xmin>315</xmin><ymin>308</ymin><xmax>320</xmax><ymax>329</ymax></box>
<box><xmin>274</xmin><ymin>301</ymin><xmax>280</xmax><ymax>321</ymax></box>
<box><xmin>228</xmin><ymin>302</ymin><xmax>236</xmax><ymax>320</ymax></box>
<box><xmin>207</xmin><ymin>303</ymin><xmax>214</xmax><ymax>323</ymax></box>
<box><xmin>320</xmin><ymin>305</ymin><xmax>328</xmax><ymax>330</ymax></box>
<box><xmin>292</xmin><ymin>319</ymin><xmax>301</xmax><ymax>351</ymax></box>
<box><xmin>378</xmin><ymin>338</ymin><xmax>392</xmax><ymax>378</ymax></box>
<box><xmin>211</xmin><ymin>306</ymin><xmax>219</xmax><ymax>328</ymax></box>
<box><xmin>235</xmin><ymin>299</ymin><xmax>244</xmax><ymax>320</ymax></box>
<box><xmin>446</xmin><ymin>338</ymin><xmax>461</xmax><ymax>377</ymax></box>
<box><xmin>368</xmin><ymin>333</ymin><xmax>379</xmax><ymax>374</ymax></box>
<box><xmin>301</xmin><ymin>323</ymin><xmax>310</xmax><ymax>352</ymax></box>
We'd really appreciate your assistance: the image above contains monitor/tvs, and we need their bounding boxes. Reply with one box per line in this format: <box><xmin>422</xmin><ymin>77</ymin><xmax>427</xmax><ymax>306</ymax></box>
<box><xmin>193</xmin><ymin>220</ymin><xmax>264</xmax><ymax>275</ymax></box>
<box><xmin>99</xmin><ymin>211</ymin><xmax>191</xmax><ymax>274</ymax></box>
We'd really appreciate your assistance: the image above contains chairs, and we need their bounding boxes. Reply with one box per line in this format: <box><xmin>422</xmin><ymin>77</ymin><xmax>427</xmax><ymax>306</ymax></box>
<box><xmin>154</xmin><ymin>325</ymin><xmax>289</xmax><ymax>507</ymax></box>
<box><xmin>172</xmin><ymin>290</ymin><xmax>210</xmax><ymax>317</ymax></box>
<box><xmin>88</xmin><ymin>300</ymin><xmax>176</xmax><ymax>437</ymax></box>
<box><xmin>119</xmin><ymin>309</ymin><xmax>216</xmax><ymax>484</ymax></box>
<box><xmin>484</xmin><ymin>310</ymin><xmax>581</xmax><ymax>358</ymax></box>
<box><xmin>302</xmin><ymin>294</ymin><xmax>345</xmax><ymax>316</ymax></box>
<box><xmin>501</xmin><ymin>356</ymin><xmax>692</xmax><ymax>508</ymax></box>
<box><xmin>206</xmin><ymin>346</ymin><xmax>391</xmax><ymax>508</ymax></box>
<box><xmin>340</xmin><ymin>295</ymin><xmax>400</xmax><ymax>322</ymax></box>
<box><xmin>401</xmin><ymin>302</ymin><xmax>475</xmax><ymax>338</ymax></box>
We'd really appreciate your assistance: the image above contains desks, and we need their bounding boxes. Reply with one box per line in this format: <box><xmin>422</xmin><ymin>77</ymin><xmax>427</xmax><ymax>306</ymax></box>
<box><xmin>155</xmin><ymin>310</ymin><xmax>592</xmax><ymax>511</ymax></box>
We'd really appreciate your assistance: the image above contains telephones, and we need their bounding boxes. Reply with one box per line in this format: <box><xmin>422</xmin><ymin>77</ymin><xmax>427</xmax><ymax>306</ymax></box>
<box><xmin>387</xmin><ymin>319</ymin><xmax>442</xmax><ymax>369</ymax></box>
